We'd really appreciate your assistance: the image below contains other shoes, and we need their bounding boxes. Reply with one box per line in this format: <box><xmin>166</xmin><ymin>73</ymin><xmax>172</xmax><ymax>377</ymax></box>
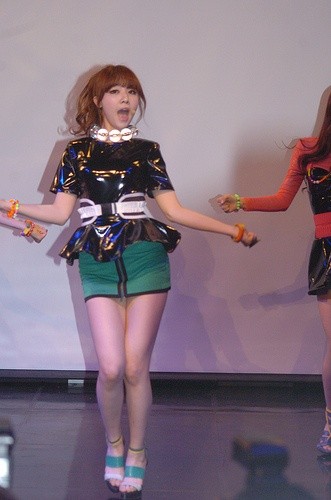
<box><xmin>317</xmin><ymin>406</ymin><xmax>331</xmax><ymax>454</ymax></box>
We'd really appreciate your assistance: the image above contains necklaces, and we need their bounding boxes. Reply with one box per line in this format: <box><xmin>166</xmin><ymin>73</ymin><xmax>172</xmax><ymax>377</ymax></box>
<box><xmin>89</xmin><ymin>124</ymin><xmax>139</xmax><ymax>144</ymax></box>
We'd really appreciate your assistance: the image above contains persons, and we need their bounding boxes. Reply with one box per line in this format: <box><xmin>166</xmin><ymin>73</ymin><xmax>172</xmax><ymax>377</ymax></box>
<box><xmin>215</xmin><ymin>92</ymin><xmax>331</xmax><ymax>459</ymax></box>
<box><xmin>1</xmin><ymin>210</ymin><xmax>48</xmax><ymax>244</ymax></box>
<box><xmin>0</xmin><ymin>65</ymin><xmax>263</xmax><ymax>499</ymax></box>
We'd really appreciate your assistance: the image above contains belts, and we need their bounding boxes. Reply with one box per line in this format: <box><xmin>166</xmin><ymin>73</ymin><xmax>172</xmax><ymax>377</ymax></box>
<box><xmin>77</xmin><ymin>200</ymin><xmax>145</xmax><ymax>219</ymax></box>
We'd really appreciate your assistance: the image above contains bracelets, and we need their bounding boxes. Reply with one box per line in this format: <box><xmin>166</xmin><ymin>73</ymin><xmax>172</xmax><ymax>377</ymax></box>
<box><xmin>13</xmin><ymin>200</ymin><xmax>19</xmax><ymax>218</ymax></box>
<box><xmin>7</xmin><ymin>200</ymin><xmax>16</xmax><ymax>218</ymax></box>
<box><xmin>25</xmin><ymin>222</ymin><xmax>35</xmax><ymax>237</ymax></box>
<box><xmin>20</xmin><ymin>220</ymin><xmax>31</xmax><ymax>236</ymax></box>
<box><xmin>231</xmin><ymin>223</ymin><xmax>245</xmax><ymax>243</ymax></box>
<box><xmin>233</xmin><ymin>193</ymin><xmax>241</xmax><ymax>213</ymax></box>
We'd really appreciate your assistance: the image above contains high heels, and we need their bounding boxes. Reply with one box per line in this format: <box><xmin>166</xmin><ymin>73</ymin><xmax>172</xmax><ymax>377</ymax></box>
<box><xmin>105</xmin><ymin>455</ymin><xmax>126</xmax><ymax>493</ymax></box>
<box><xmin>120</xmin><ymin>458</ymin><xmax>148</xmax><ymax>500</ymax></box>
